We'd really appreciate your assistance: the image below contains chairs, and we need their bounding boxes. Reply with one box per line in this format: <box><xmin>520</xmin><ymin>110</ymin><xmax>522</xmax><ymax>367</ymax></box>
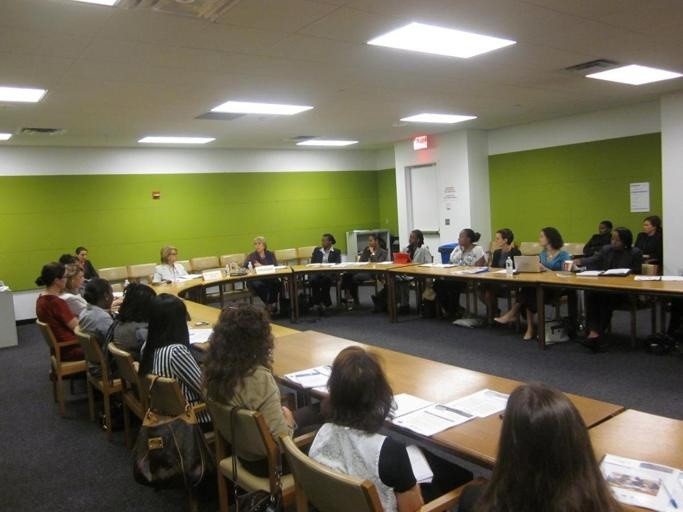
<box><xmin>204</xmin><ymin>395</ymin><xmax>320</xmax><ymax>512</ymax></box>
<box><xmin>387</xmin><ymin>239</ymin><xmax>683</xmax><ymax>350</ymax></box>
<box><xmin>35</xmin><ymin>318</ymin><xmax>88</xmax><ymax>418</ymax></box>
<box><xmin>94</xmin><ymin>247</ymin><xmax>416</xmax><ymax>325</ymax></box>
<box><xmin>282</xmin><ymin>427</ymin><xmax>487</xmax><ymax>511</ymax></box>
<box><xmin>131</xmin><ymin>361</ymin><xmax>231</xmax><ymax>503</ymax></box>
<box><xmin>108</xmin><ymin>342</ymin><xmax>149</xmax><ymax>452</ymax></box>
<box><xmin>73</xmin><ymin>324</ymin><xmax>124</xmax><ymax>441</ymax></box>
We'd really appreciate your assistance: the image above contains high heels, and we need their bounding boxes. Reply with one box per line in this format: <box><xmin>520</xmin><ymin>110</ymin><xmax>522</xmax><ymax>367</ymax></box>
<box><xmin>524</xmin><ymin>329</ymin><xmax>534</xmax><ymax>340</ymax></box>
<box><xmin>493</xmin><ymin>315</ymin><xmax>516</xmax><ymax>327</ymax></box>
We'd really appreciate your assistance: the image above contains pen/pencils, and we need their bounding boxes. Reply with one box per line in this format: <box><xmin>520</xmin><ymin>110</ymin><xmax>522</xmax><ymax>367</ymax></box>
<box><xmin>424</xmin><ymin>410</ymin><xmax>454</xmax><ymax>422</ymax></box>
<box><xmin>297</xmin><ymin>372</ymin><xmax>321</xmax><ymax>376</ymax></box>
<box><xmin>662</xmin><ymin>480</ymin><xmax>678</xmax><ymax>510</ymax></box>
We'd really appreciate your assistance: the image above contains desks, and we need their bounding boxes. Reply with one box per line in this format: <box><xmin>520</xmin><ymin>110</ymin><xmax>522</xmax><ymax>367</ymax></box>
<box><xmin>264</xmin><ymin>329</ymin><xmax>625</xmax><ymax>484</ymax></box>
<box><xmin>588</xmin><ymin>410</ymin><xmax>683</xmax><ymax>511</ymax></box>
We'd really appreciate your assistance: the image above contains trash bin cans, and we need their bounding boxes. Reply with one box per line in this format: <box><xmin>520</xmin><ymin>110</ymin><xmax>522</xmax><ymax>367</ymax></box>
<box><xmin>438</xmin><ymin>244</ymin><xmax>458</xmax><ymax>264</ymax></box>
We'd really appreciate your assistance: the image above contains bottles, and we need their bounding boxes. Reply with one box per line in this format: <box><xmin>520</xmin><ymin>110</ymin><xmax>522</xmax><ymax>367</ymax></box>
<box><xmin>123</xmin><ymin>279</ymin><xmax>129</xmax><ymax>296</ymax></box>
<box><xmin>505</xmin><ymin>256</ymin><xmax>514</xmax><ymax>277</ymax></box>
<box><xmin>247</xmin><ymin>261</ymin><xmax>252</xmax><ymax>274</ymax></box>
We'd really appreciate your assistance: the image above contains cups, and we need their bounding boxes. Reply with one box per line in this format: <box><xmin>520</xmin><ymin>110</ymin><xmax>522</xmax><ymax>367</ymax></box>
<box><xmin>225</xmin><ymin>264</ymin><xmax>231</xmax><ymax>279</ymax></box>
<box><xmin>564</xmin><ymin>260</ymin><xmax>573</xmax><ymax>272</ymax></box>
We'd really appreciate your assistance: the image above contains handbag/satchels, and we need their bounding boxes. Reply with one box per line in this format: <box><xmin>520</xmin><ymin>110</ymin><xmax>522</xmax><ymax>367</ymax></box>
<box><xmin>132</xmin><ymin>408</ymin><xmax>216</xmax><ymax>488</ymax></box>
<box><xmin>231</xmin><ymin>481</ymin><xmax>282</xmax><ymax>512</ymax></box>
<box><xmin>565</xmin><ymin>315</ymin><xmax>589</xmax><ymax>338</ymax></box>
<box><xmin>545</xmin><ymin>320</ymin><xmax>570</xmax><ymax>346</ymax></box>
<box><xmin>453</xmin><ymin>318</ymin><xmax>480</xmax><ymax>328</ymax></box>
